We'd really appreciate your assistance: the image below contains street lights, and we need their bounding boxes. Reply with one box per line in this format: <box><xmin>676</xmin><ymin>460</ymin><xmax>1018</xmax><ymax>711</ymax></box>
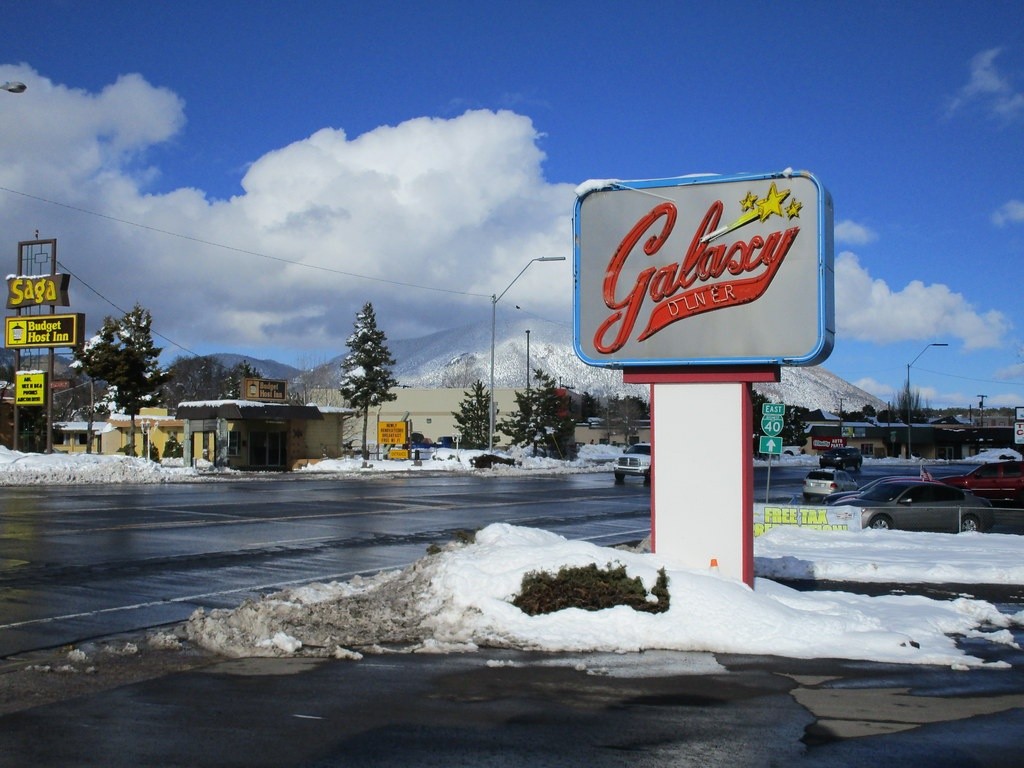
<box><xmin>906</xmin><ymin>343</ymin><xmax>948</xmax><ymax>458</ymax></box>
<box><xmin>140</xmin><ymin>419</ymin><xmax>159</xmax><ymax>461</ymax></box>
<box><xmin>488</xmin><ymin>254</ymin><xmax>566</xmax><ymax>452</ymax></box>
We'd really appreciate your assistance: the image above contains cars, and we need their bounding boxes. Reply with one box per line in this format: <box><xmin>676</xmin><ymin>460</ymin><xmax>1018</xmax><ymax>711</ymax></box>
<box><xmin>937</xmin><ymin>461</ymin><xmax>1024</xmax><ymax>509</ymax></box>
<box><xmin>822</xmin><ymin>475</ymin><xmax>997</xmax><ymax>534</ymax></box>
<box><xmin>802</xmin><ymin>468</ymin><xmax>861</xmax><ymax>502</ymax></box>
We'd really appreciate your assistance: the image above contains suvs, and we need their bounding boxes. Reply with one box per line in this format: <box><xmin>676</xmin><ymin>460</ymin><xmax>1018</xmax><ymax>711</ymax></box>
<box><xmin>614</xmin><ymin>442</ymin><xmax>651</xmax><ymax>485</ymax></box>
<box><xmin>819</xmin><ymin>446</ymin><xmax>863</xmax><ymax>471</ymax></box>
